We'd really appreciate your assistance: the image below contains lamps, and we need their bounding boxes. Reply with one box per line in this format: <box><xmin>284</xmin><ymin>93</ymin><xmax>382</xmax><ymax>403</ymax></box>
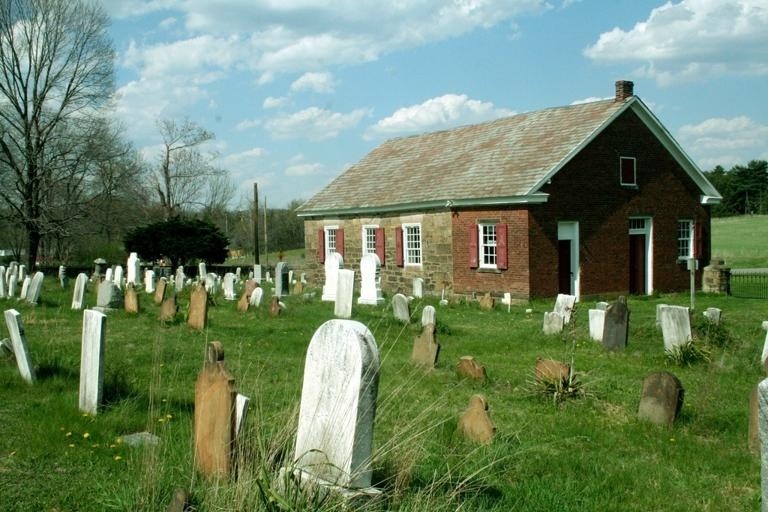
<box><xmin>445</xmin><ymin>199</ymin><xmax>454</xmax><ymax>208</ymax></box>
